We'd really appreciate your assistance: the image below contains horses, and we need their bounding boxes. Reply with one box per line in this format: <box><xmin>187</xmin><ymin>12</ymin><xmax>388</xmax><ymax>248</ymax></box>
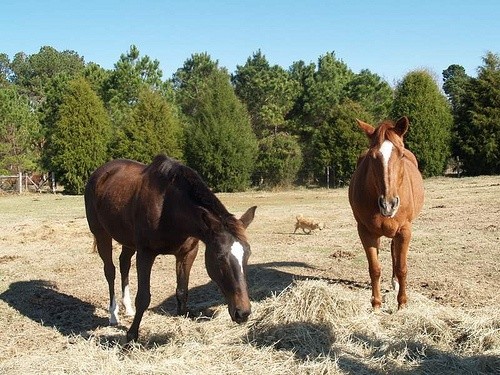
<box><xmin>84</xmin><ymin>154</ymin><xmax>258</xmax><ymax>344</ymax></box>
<box><xmin>349</xmin><ymin>116</ymin><xmax>425</xmax><ymax>311</ymax></box>
<box><xmin>25</xmin><ymin>173</ymin><xmax>47</xmax><ymax>191</ymax></box>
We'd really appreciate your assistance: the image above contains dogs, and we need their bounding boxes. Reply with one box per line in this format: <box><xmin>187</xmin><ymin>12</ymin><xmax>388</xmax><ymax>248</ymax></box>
<box><xmin>294</xmin><ymin>214</ymin><xmax>324</xmax><ymax>235</ymax></box>
